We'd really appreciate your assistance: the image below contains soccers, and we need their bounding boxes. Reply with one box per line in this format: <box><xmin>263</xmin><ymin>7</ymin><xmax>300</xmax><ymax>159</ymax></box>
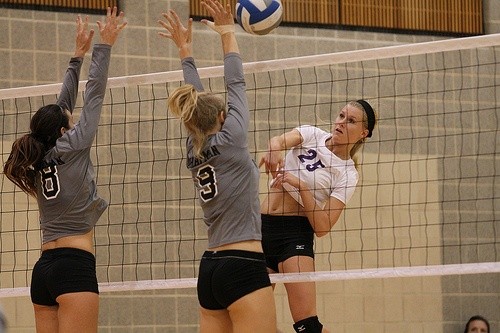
<box><xmin>236</xmin><ymin>0</ymin><xmax>283</xmax><ymax>36</ymax></box>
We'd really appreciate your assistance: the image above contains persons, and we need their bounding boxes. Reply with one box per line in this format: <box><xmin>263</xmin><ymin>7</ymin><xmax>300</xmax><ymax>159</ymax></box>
<box><xmin>3</xmin><ymin>6</ymin><xmax>128</xmax><ymax>333</ymax></box>
<box><xmin>157</xmin><ymin>0</ymin><xmax>277</xmax><ymax>333</ymax></box>
<box><xmin>464</xmin><ymin>316</ymin><xmax>490</xmax><ymax>333</ymax></box>
<box><xmin>258</xmin><ymin>99</ymin><xmax>377</xmax><ymax>333</ymax></box>
